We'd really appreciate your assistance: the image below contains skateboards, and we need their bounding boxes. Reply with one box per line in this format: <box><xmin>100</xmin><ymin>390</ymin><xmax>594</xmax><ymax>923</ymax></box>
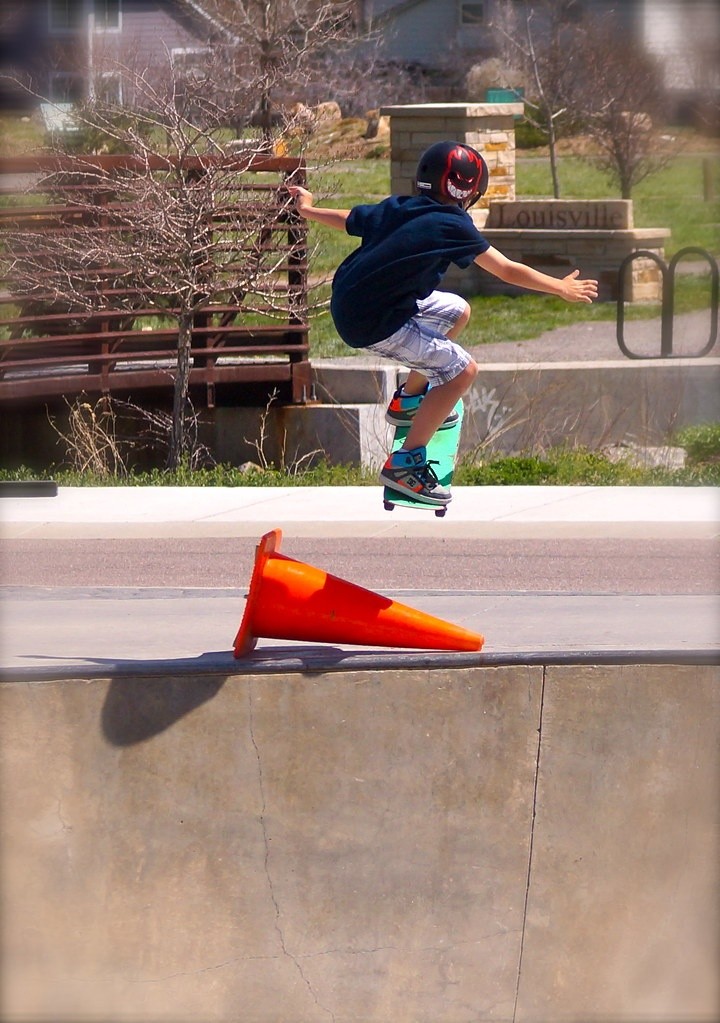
<box><xmin>381</xmin><ymin>381</ymin><xmax>465</xmax><ymax>519</ymax></box>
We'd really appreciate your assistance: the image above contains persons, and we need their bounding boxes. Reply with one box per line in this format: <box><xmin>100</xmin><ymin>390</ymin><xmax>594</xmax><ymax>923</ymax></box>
<box><xmin>287</xmin><ymin>141</ymin><xmax>600</xmax><ymax>506</ymax></box>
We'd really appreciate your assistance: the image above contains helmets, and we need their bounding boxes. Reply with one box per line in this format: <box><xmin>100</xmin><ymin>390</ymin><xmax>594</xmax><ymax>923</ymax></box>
<box><xmin>417</xmin><ymin>140</ymin><xmax>489</xmax><ymax>210</ymax></box>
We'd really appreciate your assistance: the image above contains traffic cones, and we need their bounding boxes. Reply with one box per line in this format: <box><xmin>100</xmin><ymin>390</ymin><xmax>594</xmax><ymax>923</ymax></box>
<box><xmin>232</xmin><ymin>528</ymin><xmax>484</xmax><ymax>659</ymax></box>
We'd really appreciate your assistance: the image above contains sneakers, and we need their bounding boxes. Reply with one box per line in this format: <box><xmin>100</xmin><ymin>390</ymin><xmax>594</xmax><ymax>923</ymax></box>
<box><xmin>385</xmin><ymin>382</ymin><xmax>459</xmax><ymax>430</ymax></box>
<box><xmin>378</xmin><ymin>445</ymin><xmax>453</xmax><ymax>505</ymax></box>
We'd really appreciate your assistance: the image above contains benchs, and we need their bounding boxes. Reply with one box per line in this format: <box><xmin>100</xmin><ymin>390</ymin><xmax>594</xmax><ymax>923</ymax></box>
<box><xmin>38</xmin><ymin>101</ymin><xmax>86</xmax><ymax>147</ymax></box>
